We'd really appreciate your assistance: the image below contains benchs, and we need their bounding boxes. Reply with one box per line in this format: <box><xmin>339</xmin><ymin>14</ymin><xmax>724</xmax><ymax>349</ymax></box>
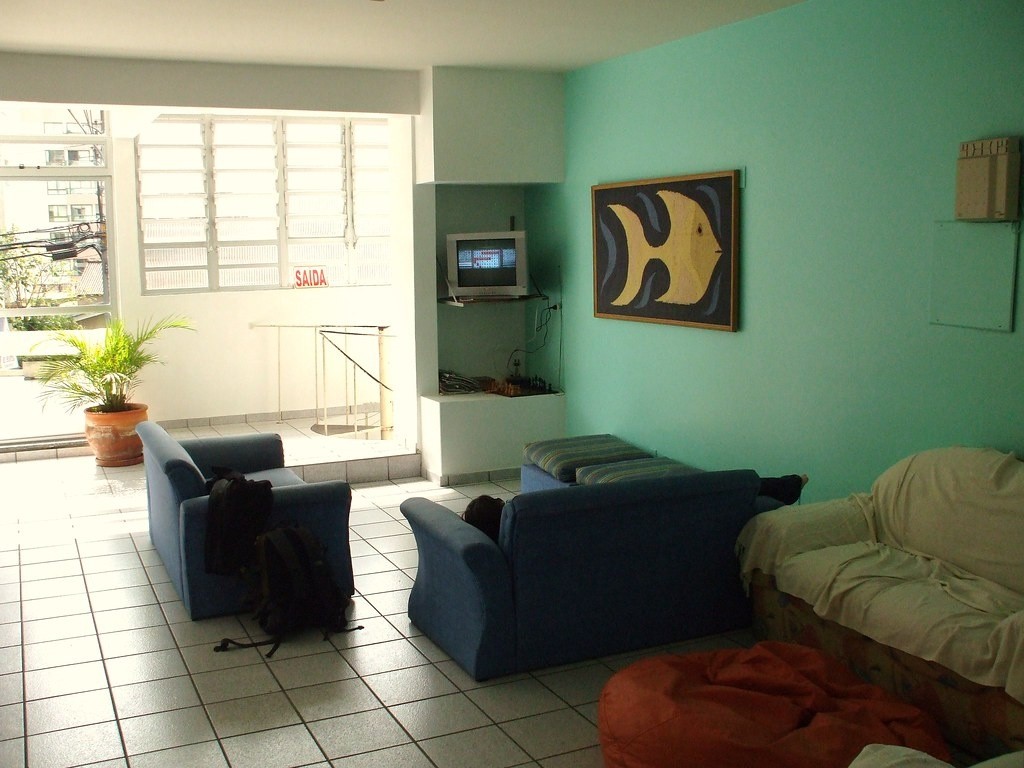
<box><xmin>520</xmin><ymin>435</ymin><xmax>703</xmax><ymax>493</ymax></box>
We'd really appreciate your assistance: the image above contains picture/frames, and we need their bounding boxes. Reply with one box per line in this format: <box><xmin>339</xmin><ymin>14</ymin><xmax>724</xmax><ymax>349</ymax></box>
<box><xmin>590</xmin><ymin>170</ymin><xmax>738</xmax><ymax>333</ymax></box>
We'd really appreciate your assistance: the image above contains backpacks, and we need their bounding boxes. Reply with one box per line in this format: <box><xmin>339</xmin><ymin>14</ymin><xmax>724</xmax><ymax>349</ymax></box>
<box><xmin>464</xmin><ymin>494</ymin><xmax>506</xmax><ymax>535</ymax></box>
<box><xmin>254</xmin><ymin>526</ymin><xmax>348</xmax><ymax>643</ymax></box>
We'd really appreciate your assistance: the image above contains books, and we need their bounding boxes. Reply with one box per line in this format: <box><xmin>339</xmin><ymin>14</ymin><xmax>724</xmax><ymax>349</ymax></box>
<box><xmin>439</xmin><ymin>369</ymin><xmax>480</xmax><ymax>396</ymax></box>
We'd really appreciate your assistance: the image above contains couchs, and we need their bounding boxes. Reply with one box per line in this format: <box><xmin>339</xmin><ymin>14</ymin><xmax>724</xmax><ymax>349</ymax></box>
<box><xmin>399</xmin><ymin>469</ymin><xmax>763</xmax><ymax>683</ymax></box>
<box><xmin>135</xmin><ymin>421</ymin><xmax>355</xmax><ymax>622</ymax></box>
<box><xmin>732</xmin><ymin>445</ymin><xmax>1024</xmax><ymax>759</ymax></box>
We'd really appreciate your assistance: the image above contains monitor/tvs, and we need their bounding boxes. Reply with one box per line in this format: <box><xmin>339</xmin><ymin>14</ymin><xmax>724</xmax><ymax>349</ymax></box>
<box><xmin>446</xmin><ymin>230</ymin><xmax>529</xmax><ymax>300</ymax></box>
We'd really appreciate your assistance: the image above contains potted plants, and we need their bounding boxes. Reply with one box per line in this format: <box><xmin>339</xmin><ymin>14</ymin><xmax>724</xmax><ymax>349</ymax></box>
<box><xmin>29</xmin><ymin>314</ymin><xmax>197</xmax><ymax>467</ymax></box>
